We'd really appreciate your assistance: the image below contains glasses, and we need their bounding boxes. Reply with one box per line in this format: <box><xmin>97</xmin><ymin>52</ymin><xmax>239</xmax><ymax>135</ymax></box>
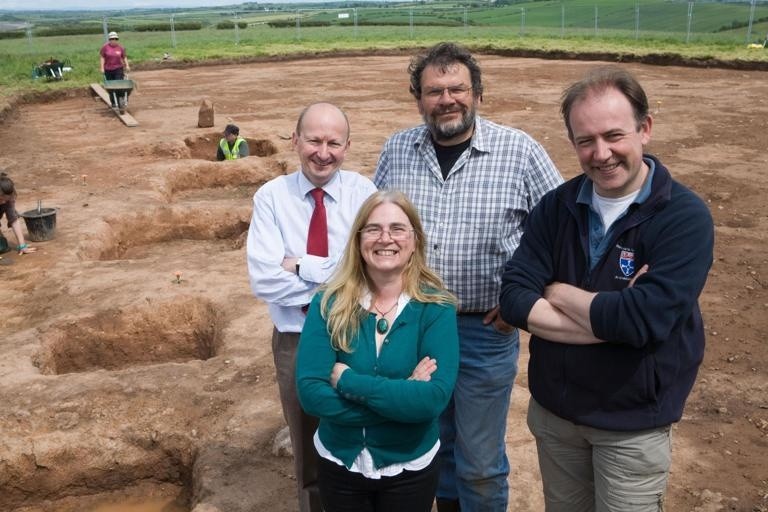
<box><xmin>356</xmin><ymin>225</ymin><xmax>417</xmax><ymax>241</ymax></box>
<box><xmin>419</xmin><ymin>84</ymin><xmax>472</xmax><ymax>103</ymax></box>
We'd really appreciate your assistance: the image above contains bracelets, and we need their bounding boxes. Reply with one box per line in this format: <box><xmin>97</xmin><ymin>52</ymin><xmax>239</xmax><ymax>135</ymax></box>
<box><xmin>296</xmin><ymin>256</ymin><xmax>302</xmax><ymax>276</ymax></box>
<box><xmin>16</xmin><ymin>244</ymin><xmax>27</xmax><ymax>250</ymax></box>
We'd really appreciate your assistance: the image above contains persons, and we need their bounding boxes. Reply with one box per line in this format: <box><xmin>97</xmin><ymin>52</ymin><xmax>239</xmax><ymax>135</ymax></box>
<box><xmin>0</xmin><ymin>171</ymin><xmax>38</xmax><ymax>260</ymax></box>
<box><xmin>217</xmin><ymin>124</ymin><xmax>250</xmax><ymax>161</ymax></box>
<box><xmin>100</xmin><ymin>31</ymin><xmax>130</xmax><ymax>107</ymax></box>
<box><xmin>372</xmin><ymin>39</ymin><xmax>563</xmax><ymax>511</ymax></box>
<box><xmin>245</xmin><ymin>101</ymin><xmax>377</xmax><ymax>511</ymax></box>
<box><xmin>295</xmin><ymin>189</ymin><xmax>462</xmax><ymax>512</ymax></box>
<box><xmin>499</xmin><ymin>64</ymin><xmax>714</xmax><ymax>512</ymax></box>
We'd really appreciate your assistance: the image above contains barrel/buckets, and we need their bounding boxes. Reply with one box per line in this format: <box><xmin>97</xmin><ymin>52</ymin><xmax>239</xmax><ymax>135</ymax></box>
<box><xmin>20</xmin><ymin>198</ymin><xmax>57</xmax><ymax>242</ymax></box>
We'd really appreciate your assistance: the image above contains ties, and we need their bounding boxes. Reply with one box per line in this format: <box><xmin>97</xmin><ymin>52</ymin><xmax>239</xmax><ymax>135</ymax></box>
<box><xmin>307</xmin><ymin>188</ymin><xmax>328</xmax><ymax>257</ymax></box>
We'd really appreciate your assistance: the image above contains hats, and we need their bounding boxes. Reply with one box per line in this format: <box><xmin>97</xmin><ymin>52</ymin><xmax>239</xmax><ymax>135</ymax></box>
<box><xmin>223</xmin><ymin>125</ymin><xmax>239</xmax><ymax>136</ymax></box>
<box><xmin>108</xmin><ymin>31</ymin><xmax>119</xmax><ymax>40</ymax></box>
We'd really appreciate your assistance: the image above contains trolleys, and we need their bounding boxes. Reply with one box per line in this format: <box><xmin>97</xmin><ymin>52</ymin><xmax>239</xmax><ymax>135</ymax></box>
<box><xmin>102</xmin><ymin>71</ymin><xmax>135</xmax><ymax>114</ymax></box>
<box><xmin>36</xmin><ymin>58</ymin><xmax>67</xmax><ymax>82</ymax></box>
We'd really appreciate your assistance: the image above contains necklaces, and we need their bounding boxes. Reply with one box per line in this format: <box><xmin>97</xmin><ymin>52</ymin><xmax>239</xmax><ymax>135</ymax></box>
<box><xmin>368</xmin><ymin>299</ymin><xmax>398</xmax><ymax>332</ymax></box>
